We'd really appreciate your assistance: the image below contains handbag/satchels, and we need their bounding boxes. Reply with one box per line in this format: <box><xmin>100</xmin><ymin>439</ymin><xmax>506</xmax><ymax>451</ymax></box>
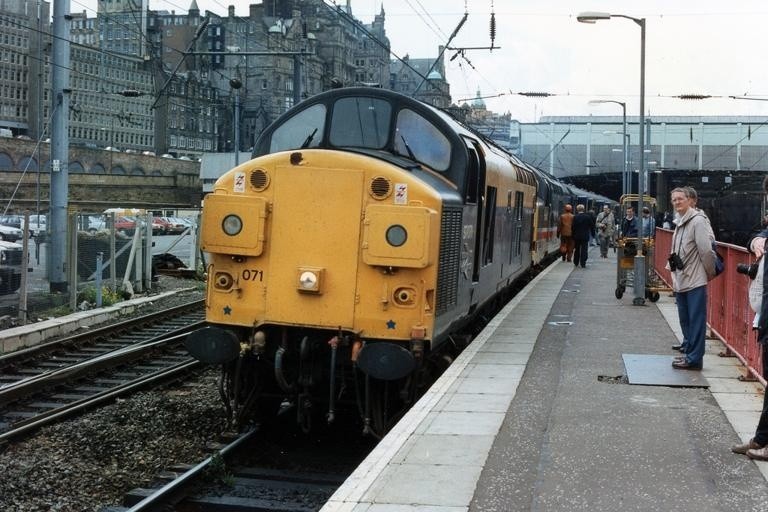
<box><xmin>713</xmin><ymin>250</ymin><xmax>725</xmax><ymax>278</ymax></box>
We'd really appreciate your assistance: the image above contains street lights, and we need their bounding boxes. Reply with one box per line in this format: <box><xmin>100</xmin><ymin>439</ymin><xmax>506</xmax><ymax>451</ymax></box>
<box><xmin>588</xmin><ymin>100</ymin><xmax>630</xmax><ymax>219</ymax></box>
<box><xmin>578</xmin><ymin>11</ymin><xmax>645</xmax><ymax>306</ymax></box>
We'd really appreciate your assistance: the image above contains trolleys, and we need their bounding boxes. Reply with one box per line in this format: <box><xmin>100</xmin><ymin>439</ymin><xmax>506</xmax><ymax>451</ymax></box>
<box><xmin>615</xmin><ymin>194</ymin><xmax>659</xmax><ymax>302</ymax></box>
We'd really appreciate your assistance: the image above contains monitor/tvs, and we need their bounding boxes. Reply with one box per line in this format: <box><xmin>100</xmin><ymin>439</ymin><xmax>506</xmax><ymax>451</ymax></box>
<box><xmin>736</xmin><ymin>263</ymin><xmax>759</xmax><ymax>279</ymax></box>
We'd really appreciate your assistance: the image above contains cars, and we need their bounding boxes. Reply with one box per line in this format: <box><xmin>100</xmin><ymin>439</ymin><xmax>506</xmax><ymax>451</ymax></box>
<box><xmin>0</xmin><ymin>215</ymin><xmax>47</xmax><ymax>292</ymax></box>
<box><xmin>78</xmin><ymin>208</ymin><xmax>197</xmax><ymax>236</ymax></box>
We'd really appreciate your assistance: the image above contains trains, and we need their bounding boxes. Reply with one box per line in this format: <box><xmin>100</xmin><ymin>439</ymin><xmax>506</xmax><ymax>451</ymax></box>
<box><xmin>185</xmin><ymin>87</ymin><xmax>618</xmax><ymax>439</ymax></box>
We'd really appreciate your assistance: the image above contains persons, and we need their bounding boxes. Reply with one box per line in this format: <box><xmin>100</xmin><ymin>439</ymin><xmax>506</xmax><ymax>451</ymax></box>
<box><xmin>620</xmin><ymin>207</ymin><xmax>640</xmax><ymax>237</ymax></box>
<box><xmin>729</xmin><ymin>210</ymin><xmax>767</xmax><ymax>461</ymax></box>
<box><xmin>595</xmin><ymin>203</ymin><xmax>616</xmax><ymax>260</ymax></box>
<box><xmin>555</xmin><ymin>204</ymin><xmax>577</xmax><ymax>262</ymax></box>
<box><xmin>641</xmin><ymin>206</ymin><xmax>657</xmax><ymax>237</ymax></box>
<box><xmin>586</xmin><ymin>209</ymin><xmax>602</xmax><ymax>246</ymax></box>
<box><xmin>671</xmin><ymin>185</ymin><xmax>716</xmax><ymax>356</ymax></box>
<box><xmin>667</xmin><ymin>186</ymin><xmax>718</xmax><ymax>370</ymax></box>
<box><xmin>570</xmin><ymin>204</ymin><xmax>595</xmax><ymax>268</ymax></box>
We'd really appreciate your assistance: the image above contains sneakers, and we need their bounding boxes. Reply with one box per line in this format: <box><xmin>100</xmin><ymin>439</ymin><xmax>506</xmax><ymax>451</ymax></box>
<box><xmin>746</xmin><ymin>445</ymin><xmax>768</xmax><ymax>460</ymax></box>
<box><xmin>731</xmin><ymin>438</ymin><xmax>763</xmax><ymax>454</ymax></box>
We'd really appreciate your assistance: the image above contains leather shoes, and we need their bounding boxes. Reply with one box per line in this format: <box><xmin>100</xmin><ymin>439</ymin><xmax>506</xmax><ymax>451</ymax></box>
<box><xmin>670</xmin><ymin>344</ymin><xmax>702</xmax><ymax>370</ymax></box>
